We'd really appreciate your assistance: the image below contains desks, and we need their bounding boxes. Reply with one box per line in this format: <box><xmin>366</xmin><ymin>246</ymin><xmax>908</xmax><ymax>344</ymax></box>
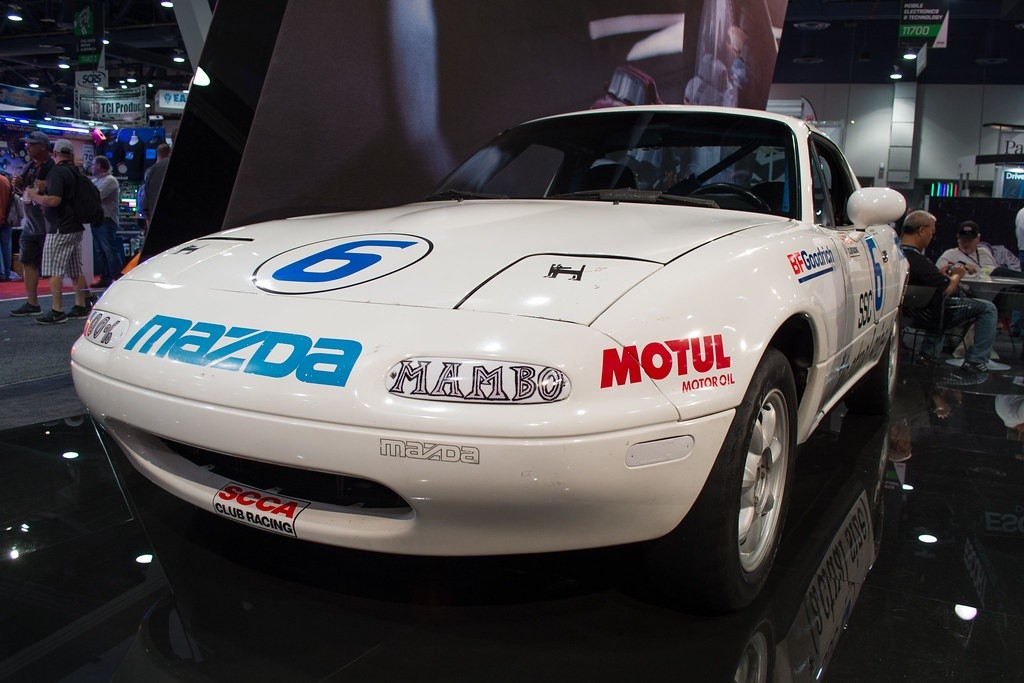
<box><xmin>955</xmin><ymin>272</ymin><xmax>1024</xmax><ymax>306</ymax></box>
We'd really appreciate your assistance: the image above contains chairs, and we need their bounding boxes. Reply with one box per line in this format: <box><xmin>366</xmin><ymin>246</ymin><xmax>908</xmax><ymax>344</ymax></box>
<box><xmin>579</xmin><ymin>163</ymin><xmax>638</xmax><ymax>190</ymax></box>
<box><xmin>902</xmin><ymin>285</ymin><xmax>968</xmax><ymax>365</ymax></box>
<box><xmin>751</xmin><ymin>180</ymin><xmax>784</xmax><ymax>212</ymax></box>
<box><xmin>989</xmin><ymin>265</ymin><xmax>1024</xmax><ymax>354</ymax></box>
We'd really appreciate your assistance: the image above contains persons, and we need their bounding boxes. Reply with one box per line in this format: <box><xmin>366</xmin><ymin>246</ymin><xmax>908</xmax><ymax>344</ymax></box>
<box><xmin>886</xmin><ymin>395</ymin><xmax>1023</xmax><ymax>461</ymax></box>
<box><xmin>898</xmin><ymin>210</ymin><xmax>998</xmax><ymax>378</ymax></box>
<box><xmin>0</xmin><ymin>130</ymin><xmax>176</xmax><ymax>324</ymax></box>
<box><xmin>935</xmin><ymin>208</ymin><xmax>1024</xmax><ymax>359</ymax></box>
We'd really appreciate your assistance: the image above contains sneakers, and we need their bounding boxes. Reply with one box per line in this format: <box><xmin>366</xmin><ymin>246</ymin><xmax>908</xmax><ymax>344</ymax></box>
<box><xmin>85</xmin><ymin>293</ymin><xmax>99</xmax><ymax>311</ymax></box>
<box><xmin>34</xmin><ymin>309</ymin><xmax>68</xmax><ymax>325</ymax></box>
<box><xmin>67</xmin><ymin>304</ymin><xmax>91</xmax><ymax>320</ymax></box>
<box><xmin>9</xmin><ymin>303</ymin><xmax>43</xmax><ymax>317</ymax></box>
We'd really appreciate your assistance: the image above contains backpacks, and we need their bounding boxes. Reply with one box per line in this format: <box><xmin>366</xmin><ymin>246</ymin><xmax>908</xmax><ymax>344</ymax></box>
<box><xmin>53</xmin><ymin>163</ymin><xmax>101</xmax><ymax>224</ymax></box>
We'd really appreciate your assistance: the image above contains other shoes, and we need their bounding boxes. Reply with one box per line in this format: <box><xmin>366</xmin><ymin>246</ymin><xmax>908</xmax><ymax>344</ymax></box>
<box><xmin>89</xmin><ymin>277</ymin><xmax>112</xmax><ymax>288</ymax></box>
<box><xmin>963</xmin><ymin>359</ymin><xmax>1011</xmax><ymax>372</ymax></box>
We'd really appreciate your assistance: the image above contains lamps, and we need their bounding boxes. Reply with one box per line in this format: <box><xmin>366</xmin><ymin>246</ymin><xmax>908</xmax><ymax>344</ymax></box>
<box><xmin>890</xmin><ymin>65</ymin><xmax>903</xmax><ymax>80</ymax></box>
<box><xmin>7</xmin><ymin>4</ymin><xmax>23</xmax><ymax>22</ymax></box>
<box><xmin>29</xmin><ymin>77</ymin><xmax>40</xmax><ymax>88</ymax></box>
<box><xmin>903</xmin><ymin>45</ymin><xmax>917</xmax><ymax>60</ymax></box>
<box><xmin>127</xmin><ymin>70</ymin><xmax>137</xmax><ymax>83</ymax></box>
<box><xmin>57</xmin><ymin>57</ymin><xmax>71</xmax><ymax>70</ymax></box>
<box><xmin>173</xmin><ymin>48</ymin><xmax>185</xmax><ymax>63</ymax></box>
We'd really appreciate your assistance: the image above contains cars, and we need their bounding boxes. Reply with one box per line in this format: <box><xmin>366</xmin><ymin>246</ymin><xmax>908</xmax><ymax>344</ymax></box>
<box><xmin>67</xmin><ymin>103</ymin><xmax>910</xmax><ymax>605</ymax></box>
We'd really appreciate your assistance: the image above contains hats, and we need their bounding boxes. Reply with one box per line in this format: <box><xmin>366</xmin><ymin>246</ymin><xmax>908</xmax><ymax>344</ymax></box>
<box><xmin>19</xmin><ymin>132</ymin><xmax>50</xmax><ymax>149</ymax></box>
<box><xmin>957</xmin><ymin>221</ymin><xmax>980</xmax><ymax>240</ymax></box>
<box><xmin>52</xmin><ymin>139</ymin><xmax>73</xmax><ymax>155</ymax></box>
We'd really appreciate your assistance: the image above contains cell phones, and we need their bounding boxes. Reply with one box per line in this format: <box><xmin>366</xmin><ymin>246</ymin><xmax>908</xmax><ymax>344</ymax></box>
<box><xmin>946</xmin><ymin>261</ymin><xmax>965</xmax><ymax>274</ymax></box>
